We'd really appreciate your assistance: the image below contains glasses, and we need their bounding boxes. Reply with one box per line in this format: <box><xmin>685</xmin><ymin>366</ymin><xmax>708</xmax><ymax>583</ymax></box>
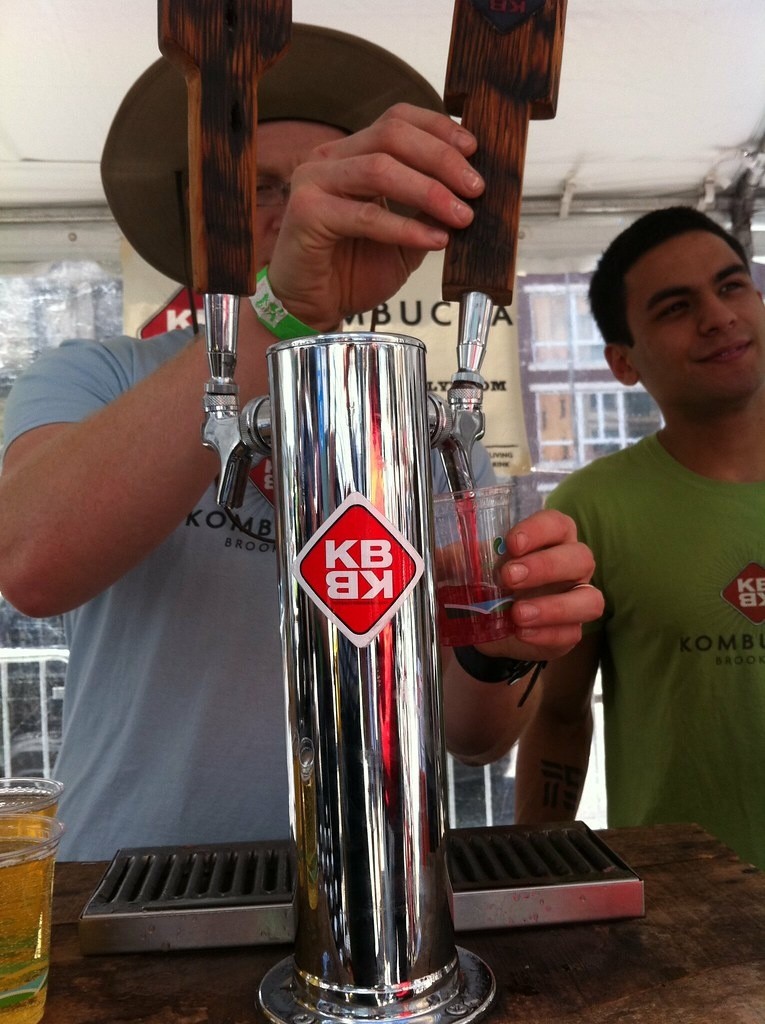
<box><xmin>254</xmin><ymin>168</ymin><xmax>289</xmax><ymax>203</ymax></box>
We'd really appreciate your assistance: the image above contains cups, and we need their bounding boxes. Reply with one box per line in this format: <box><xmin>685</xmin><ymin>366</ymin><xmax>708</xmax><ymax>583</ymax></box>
<box><xmin>429</xmin><ymin>483</ymin><xmax>545</xmax><ymax>648</ymax></box>
<box><xmin>0</xmin><ymin>776</ymin><xmax>67</xmax><ymax>1024</ymax></box>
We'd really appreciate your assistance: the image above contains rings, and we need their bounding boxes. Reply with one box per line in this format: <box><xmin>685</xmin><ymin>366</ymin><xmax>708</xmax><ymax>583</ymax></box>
<box><xmin>566</xmin><ymin>582</ymin><xmax>595</xmax><ymax>595</ymax></box>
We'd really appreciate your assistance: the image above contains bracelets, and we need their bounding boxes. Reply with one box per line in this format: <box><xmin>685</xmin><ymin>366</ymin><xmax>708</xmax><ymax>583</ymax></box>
<box><xmin>455</xmin><ymin>642</ymin><xmax>546</xmax><ymax>704</ymax></box>
<box><xmin>249</xmin><ymin>268</ymin><xmax>315</xmax><ymax>337</ymax></box>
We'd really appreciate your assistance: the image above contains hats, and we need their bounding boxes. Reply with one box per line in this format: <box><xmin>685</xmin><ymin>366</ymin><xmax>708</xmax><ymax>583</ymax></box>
<box><xmin>100</xmin><ymin>21</ymin><xmax>450</xmax><ymax>289</ymax></box>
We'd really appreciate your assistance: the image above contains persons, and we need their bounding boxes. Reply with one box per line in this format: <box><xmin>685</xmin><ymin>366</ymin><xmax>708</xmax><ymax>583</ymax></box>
<box><xmin>516</xmin><ymin>205</ymin><xmax>765</xmax><ymax>870</ymax></box>
<box><xmin>0</xmin><ymin>21</ymin><xmax>603</xmax><ymax>863</ymax></box>
